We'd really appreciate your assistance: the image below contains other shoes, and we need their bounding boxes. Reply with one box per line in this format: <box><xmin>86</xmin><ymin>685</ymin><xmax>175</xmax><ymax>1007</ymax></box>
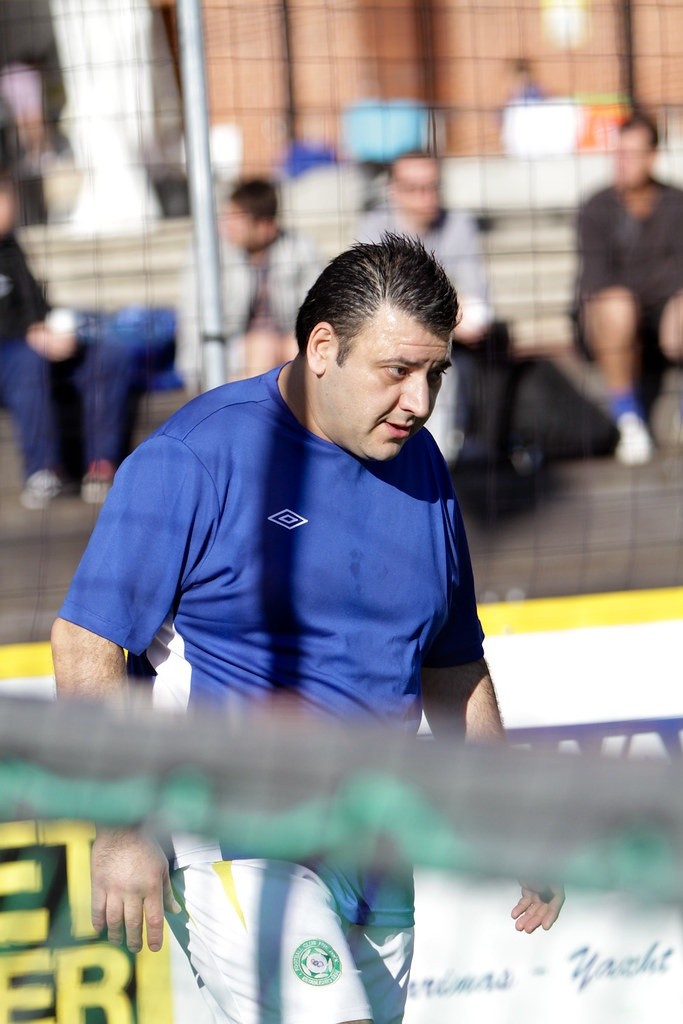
<box><xmin>80</xmin><ymin>476</ymin><xmax>111</xmax><ymax>506</ymax></box>
<box><xmin>617</xmin><ymin>415</ymin><xmax>654</xmax><ymax>469</ymax></box>
<box><xmin>19</xmin><ymin>469</ymin><xmax>60</xmax><ymax>511</ymax></box>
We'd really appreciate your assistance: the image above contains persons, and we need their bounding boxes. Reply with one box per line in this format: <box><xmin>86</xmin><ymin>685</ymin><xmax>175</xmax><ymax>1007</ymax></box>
<box><xmin>0</xmin><ymin>178</ymin><xmax>130</xmax><ymax>508</ymax></box>
<box><xmin>221</xmin><ymin>180</ymin><xmax>320</xmax><ymax>380</ymax></box>
<box><xmin>355</xmin><ymin>152</ymin><xmax>518</xmax><ymax>503</ymax></box>
<box><xmin>568</xmin><ymin>117</ymin><xmax>683</xmax><ymax>464</ymax></box>
<box><xmin>51</xmin><ymin>237</ymin><xmax>564</xmax><ymax>1024</ymax></box>
